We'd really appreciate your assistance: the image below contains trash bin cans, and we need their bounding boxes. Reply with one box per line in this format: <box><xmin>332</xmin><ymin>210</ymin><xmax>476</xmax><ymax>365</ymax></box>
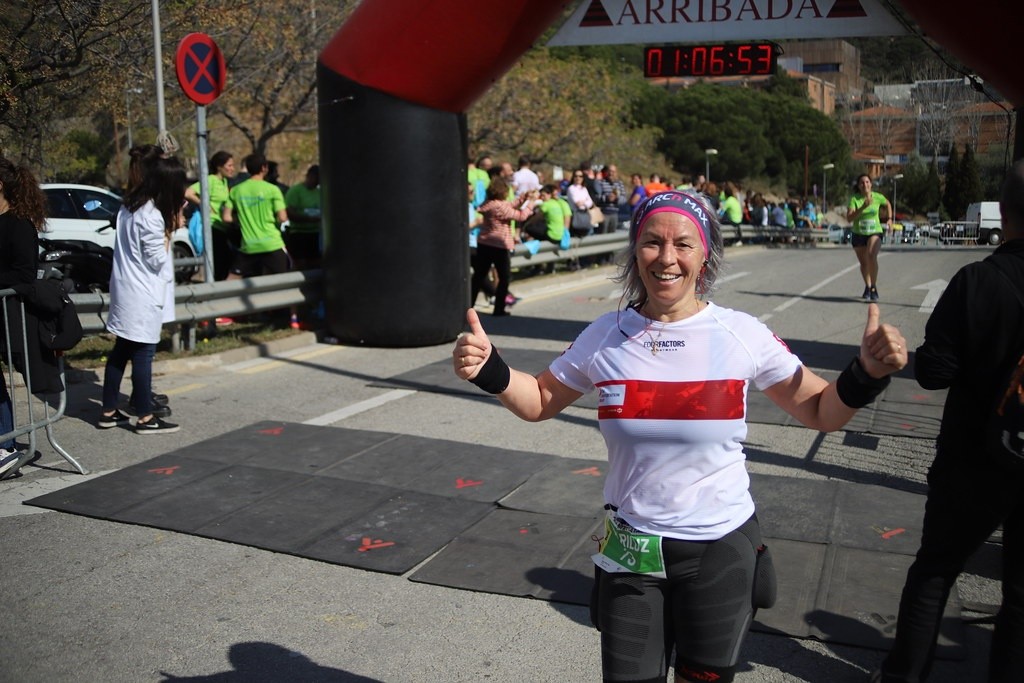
<box><xmin>881</xmin><ymin>223</ymin><xmax>903</xmax><ymax>244</ymax></box>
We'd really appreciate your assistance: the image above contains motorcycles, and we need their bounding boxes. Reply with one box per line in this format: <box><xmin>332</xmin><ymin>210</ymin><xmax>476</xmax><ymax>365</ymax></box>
<box><xmin>38</xmin><ymin>200</ymin><xmax>118</xmax><ymax>294</ymax></box>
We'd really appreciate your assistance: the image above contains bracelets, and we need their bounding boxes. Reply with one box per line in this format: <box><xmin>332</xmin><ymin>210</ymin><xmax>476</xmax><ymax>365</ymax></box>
<box><xmin>887</xmin><ymin>217</ymin><xmax>892</xmax><ymax>219</ymax></box>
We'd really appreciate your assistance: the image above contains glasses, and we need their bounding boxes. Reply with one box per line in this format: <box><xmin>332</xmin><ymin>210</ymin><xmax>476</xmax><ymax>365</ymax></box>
<box><xmin>574</xmin><ymin>175</ymin><xmax>584</xmax><ymax>178</ymax></box>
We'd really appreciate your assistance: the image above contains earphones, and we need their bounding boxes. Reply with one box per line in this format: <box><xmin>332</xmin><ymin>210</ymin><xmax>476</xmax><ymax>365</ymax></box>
<box><xmin>701</xmin><ymin>260</ymin><xmax>709</xmax><ymax>275</ymax></box>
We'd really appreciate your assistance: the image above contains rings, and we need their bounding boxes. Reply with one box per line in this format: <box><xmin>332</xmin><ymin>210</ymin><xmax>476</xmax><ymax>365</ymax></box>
<box><xmin>898</xmin><ymin>345</ymin><xmax>901</xmax><ymax>352</ymax></box>
<box><xmin>461</xmin><ymin>357</ymin><xmax>465</xmax><ymax>366</ymax></box>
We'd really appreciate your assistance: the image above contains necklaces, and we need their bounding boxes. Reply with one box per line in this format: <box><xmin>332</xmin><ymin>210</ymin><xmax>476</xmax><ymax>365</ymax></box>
<box><xmin>642</xmin><ymin>297</ymin><xmax>700</xmax><ymax>356</ymax></box>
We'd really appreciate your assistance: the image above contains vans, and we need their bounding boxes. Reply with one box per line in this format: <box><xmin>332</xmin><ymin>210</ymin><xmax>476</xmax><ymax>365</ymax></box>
<box><xmin>966</xmin><ymin>201</ymin><xmax>1003</xmax><ymax>245</ymax></box>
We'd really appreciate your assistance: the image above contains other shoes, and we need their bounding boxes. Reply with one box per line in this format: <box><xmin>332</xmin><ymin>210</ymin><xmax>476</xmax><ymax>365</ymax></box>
<box><xmin>731</xmin><ymin>240</ymin><xmax>743</xmax><ymax>247</ymax></box>
<box><xmin>131</xmin><ymin>394</ymin><xmax>171</xmax><ymax>417</ymax></box>
<box><xmin>492</xmin><ymin>311</ymin><xmax>511</xmax><ymax>317</ymax></box>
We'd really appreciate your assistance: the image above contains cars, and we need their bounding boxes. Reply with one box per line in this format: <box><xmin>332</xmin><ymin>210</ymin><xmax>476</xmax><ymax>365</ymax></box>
<box><xmin>920</xmin><ymin>223</ymin><xmax>944</xmax><ymax>236</ymax></box>
<box><xmin>819</xmin><ymin>223</ymin><xmax>844</xmax><ymax>243</ymax></box>
<box><xmin>36</xmin><ymin>182</ymin><xmax>202</xmax><ymax>280</ymax></box>
<box><xmin>841</xmin><ymin>225</ymin><xmax>920</xmax><ymax>243</ymax></box>
<box><xmin>873</xmin><ymin>177</ymin><xmax>884</xmax><ymax>187</ymax></box>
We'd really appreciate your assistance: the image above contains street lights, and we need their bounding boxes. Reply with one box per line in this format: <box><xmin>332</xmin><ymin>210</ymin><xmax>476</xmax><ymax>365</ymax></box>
<box><xmin>706</xmin><ymin>148</ymin><xmax>718</xmax><ymax>181</ymax></box>
<box><xmin>892</xmin><ymin>173</ymin><xmax>903</xmax><ymax>224</ymax></box>
<box><xmin>823</xmin><ymin>163</ymin><xmax>835</xmax><ymax>214</ymax></box>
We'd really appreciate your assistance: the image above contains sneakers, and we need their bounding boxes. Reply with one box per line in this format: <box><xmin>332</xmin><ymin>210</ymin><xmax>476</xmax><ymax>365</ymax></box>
<box><xmin>135</xmin><ymin>413</ymin><xmax>181</xmax><ymax>435</ymax></box>
<box><xmin>97</xmin><ymin>409</ymin><xmax>132</xmax><ymax>428</ymax></box>
<box><xmin>200</xmin><ymin>316</ymin><xmax>233</xmax><ymax>328</ymax></box>
<box><xmin>870</xmin><ymin>285</ymin><xmax>879</xmax><ymax>303</ymax></box>
<box><xmin>287</xmin><ymin>318</ymin><xmax>317</xmax><ymax>332</ymax></box>
<box><xmin>862</xmin><ymin>287</ymin><xmax>871</xmax><ymax>299</ymax></box>
<box><xmin>0</xmin><ymin>447</ymin><xmax>21</xmax><ymax>478</ymax></box>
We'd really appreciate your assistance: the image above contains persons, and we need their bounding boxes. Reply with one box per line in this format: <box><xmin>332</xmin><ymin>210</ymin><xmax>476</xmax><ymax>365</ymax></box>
<box><xmin>454</xmin><ymin>191</ymin><xmax>909</xmax><ymax>683</ymax></box>
<box><xmin>0</xmin><ymin>148</ymin><xmax>46</xmax><ymax>476</ymax></box>
<box><xmin>48</xmin><ymin>144</ymin><xmax>186</xmax><ymax>435</ymax></box>
<box><xmin>469</xmin><ymin>149</ymin><xmax>826</xmax><ymax>319</ymax></box>
<box><xmin>882</xmin><ymin>158</ymin><xmax>1024</xmax><ymax>683</ymax></box>
<box><xmin>846</xmin><ymin>174</ymin><xmax>894</xmax><ymax>300</ymax></box>
<box><xmin>185</xmin><ymin>152</ymin><xmax>322</xmax><ymax>328</ymax></box>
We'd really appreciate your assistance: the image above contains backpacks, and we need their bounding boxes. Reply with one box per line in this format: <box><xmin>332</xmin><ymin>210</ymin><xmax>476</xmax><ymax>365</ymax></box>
<box><xmin>955</xmin><ymin>252</ymin><xmax>1024</xmax><ymax>493</ymax></box>
